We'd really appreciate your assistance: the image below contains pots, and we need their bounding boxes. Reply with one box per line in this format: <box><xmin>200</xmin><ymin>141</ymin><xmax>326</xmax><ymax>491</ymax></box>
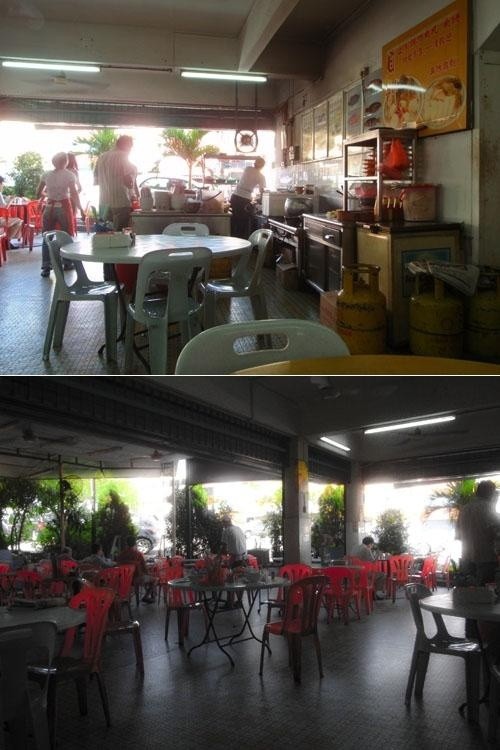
<box><xmin>283</xmin><ymin>197</ymin><xmax>315</xmax><ymax>217</ymax></box>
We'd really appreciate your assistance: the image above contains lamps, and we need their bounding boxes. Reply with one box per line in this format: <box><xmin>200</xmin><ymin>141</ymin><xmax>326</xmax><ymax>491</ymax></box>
<box><xmin>321</xmin><ymin>437</ymin><xmax>353</xmax><ymax>453</ymax></box>
<box><xmin>177</xmin><ymin>69</ymin><xmax>271</xmax><ymax>86</ymax></box>
<box><xmin>0</xmin><ymin>56</ymin><xmax>101</xmax><ymax>77</ymax></box>
<box><xmin>363</xmin><ymin>415</ymin><xmax>456</xmax><ymax>436</ymax></box>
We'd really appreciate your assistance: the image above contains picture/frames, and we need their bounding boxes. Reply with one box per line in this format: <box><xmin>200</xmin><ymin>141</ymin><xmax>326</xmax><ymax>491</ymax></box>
<box><xmin>298</xmin><ymin>67</ymin><xmax>384</xmax><ymax>166</ymax></box>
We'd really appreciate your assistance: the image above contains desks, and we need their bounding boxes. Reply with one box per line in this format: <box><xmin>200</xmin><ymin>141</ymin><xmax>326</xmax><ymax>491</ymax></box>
<box><xmin>230</xmin><ymin>354</ymin><xmax>499</xmax><ymax>376</ymax></box>
<box><xmin>59</xmin><ymin>234</ymin><xmax>254</xmax><ymax>374</ymax></box>
<box><xmin>418</xmin><ymin>591</ymin><xmax>500</xmax><ymax>724</ymax></box>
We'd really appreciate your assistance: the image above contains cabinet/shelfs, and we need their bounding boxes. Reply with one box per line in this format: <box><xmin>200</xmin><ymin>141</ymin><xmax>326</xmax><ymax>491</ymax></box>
<box><xmin>338</xmin><ymin>125</ymin><xmax>422</xmax><ymax>221</ymax></box>
<box><xmin>302</xmin><ymin>209</ymin><xmax>357</xmax><ymax>300</ymax></box>
<box><xmin>355</xmin><ymin>219</ymin><xmax>464</xmax><ymax>342</ymax></box>
<box><xmin>265</xmin><ymin>216</ymin><xmax>303</xmax><ymax>277</ymax></box>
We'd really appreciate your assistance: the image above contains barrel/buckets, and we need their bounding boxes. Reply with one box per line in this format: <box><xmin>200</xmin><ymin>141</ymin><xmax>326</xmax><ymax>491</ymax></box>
<box><xmin>201</xmin><ymin>189</ymin><xmax>223</xmax><ymax>215</ymax></box>
<box><xmin>399</xmin><ymin>185</ymin><xmax>437</xmax><ymax>222</ymax></box>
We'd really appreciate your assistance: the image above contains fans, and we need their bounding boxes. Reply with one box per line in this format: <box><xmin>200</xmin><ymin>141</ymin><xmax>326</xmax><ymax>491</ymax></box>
<box><xmin>395</xmin><ymin>426</ymin><xmax>470</xmax><ymax>450</ymax></box>
<box><xmin>235</xmin><ymin>128</ymin><xmax>258</xmax><ymax>154</ymax></box>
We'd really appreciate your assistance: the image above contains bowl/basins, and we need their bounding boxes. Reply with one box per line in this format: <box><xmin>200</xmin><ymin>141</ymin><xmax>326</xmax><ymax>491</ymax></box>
<box><xmin>183</xmin><ymin>202</ymin><xmax>201</xmax><ymax>213</ymax></box>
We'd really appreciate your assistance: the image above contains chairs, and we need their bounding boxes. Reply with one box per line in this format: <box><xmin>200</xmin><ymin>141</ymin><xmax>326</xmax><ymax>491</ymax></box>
<box><xmin>197</xmin><ymin>225</ymin><xmax>276</xmax><ymax>350</ymax></box>
<box><xmin>400</xmin><ymin>581</ymin><xmax>484</xmax><ymax>726</ymax></box>
<box><xmin>40</xmin><ymin>229</ymin><xmax>129</xmax><ymax>365</ymax></box>
<box><xmin>115</xmin><ymin>246</ymin><xmax>213</xmax><ymax>375</ymax></box>
<box><xmin>0</xmin><ymin>197</ymin><xmax>77</xmax><ymax>269</ymax></box>
<box><xmin>143</xmin><ymin>222</ymin><xmax>211</xmax><ymax>337</ymax></box>
<box><xmin>174</xmin><ymin>319</ymin><xmax>353</xmax><ymax>376</ymax></box>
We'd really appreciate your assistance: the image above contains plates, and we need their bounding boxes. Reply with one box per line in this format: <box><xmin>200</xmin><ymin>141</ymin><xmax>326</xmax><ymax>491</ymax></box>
<box><xmin>382</xmin><ymin>75</ymin><xmax>466</xmax><ymax>130</ymax></box>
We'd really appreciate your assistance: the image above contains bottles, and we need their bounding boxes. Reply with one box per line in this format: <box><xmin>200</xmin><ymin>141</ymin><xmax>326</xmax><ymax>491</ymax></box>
<box><xmin>373</xmin><ymin>195</ymin><xmax>403</xmax><ymax>224</ymax></box>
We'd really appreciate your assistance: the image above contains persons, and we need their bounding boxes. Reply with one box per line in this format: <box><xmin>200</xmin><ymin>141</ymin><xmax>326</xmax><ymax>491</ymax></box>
<box><xmin>0</xmin><ymin>480</ymin><xmax>500</xmax><ymax>645</ymax></box>
<box><xmin>0</xmin><ymin>135</ymin><xmax>267</xmax><ymax>278</ymax></box>
